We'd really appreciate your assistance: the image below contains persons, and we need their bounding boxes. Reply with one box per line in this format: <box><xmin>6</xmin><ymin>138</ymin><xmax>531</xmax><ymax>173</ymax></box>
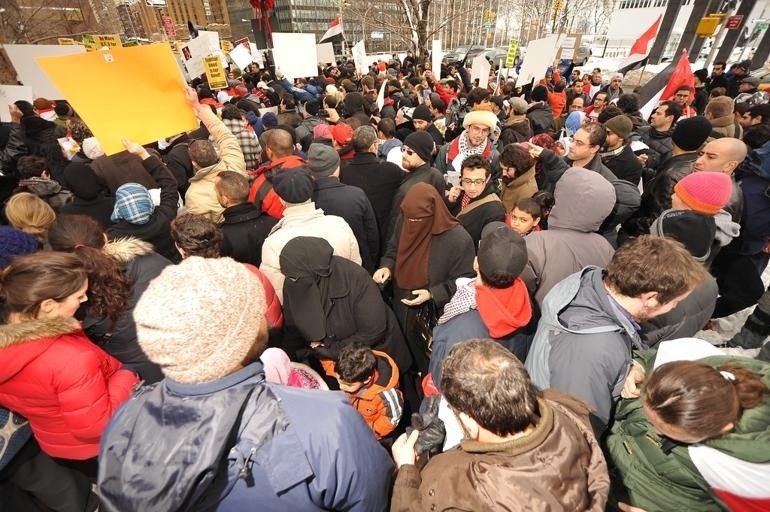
<box><xmin>0</xmin><ymin>50</ymin><xmax>769</xmax><ymax>512</ymax></box>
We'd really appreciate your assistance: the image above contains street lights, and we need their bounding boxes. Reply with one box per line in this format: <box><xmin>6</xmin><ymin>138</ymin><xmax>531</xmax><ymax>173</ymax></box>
<box><xmin>345</xmin><ymin>3</ymin><xmax>380</xmax><ymax>42</ymax></box>
<box><xmin>379</xmin><ymin>12</ymin><xmax>392</xmax><ymax>53</ymax></box>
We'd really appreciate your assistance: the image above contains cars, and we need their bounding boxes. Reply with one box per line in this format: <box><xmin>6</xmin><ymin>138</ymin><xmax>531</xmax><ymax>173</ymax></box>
<box><xmin>442</xmin><ymin>46</ymin><xmax>521</xmax><ymax>70</ymax></box>
<box><xmin>574</xmin><ymin>44</ymin><xmax>592</xmax><ymax>66</ymax></box>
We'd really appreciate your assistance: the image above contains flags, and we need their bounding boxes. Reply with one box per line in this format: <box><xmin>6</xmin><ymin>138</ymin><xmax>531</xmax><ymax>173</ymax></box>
<box><xmin>628</xmin><ymin>14</ymin><xmax>663</xmax><ymax>57</ymax></box>
<box><xmin>319</xmin><ymin>17</ymin><xmax>345</xmax><ymax>44</ymax></box>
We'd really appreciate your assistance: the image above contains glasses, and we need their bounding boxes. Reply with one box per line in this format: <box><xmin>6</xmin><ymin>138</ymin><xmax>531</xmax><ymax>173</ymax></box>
<box><xmin>472</xmin><ymin>126</ymin><xmax>489</xmax><ymax>132</ymax></box>
<box><xmin>401</xmin><ymin>148</ymin><xmax>416</xmax><ymax>155</ymax></box>
<box><xmin>568</xmin><ymin>138</ymin><xmax>593</xmax><ymax>146</ymax></box>
<box><xmin>595</xmin><ymin>99</ymin><xmax>604</xmax><ymax>101</ymax></box>
<box><xmin>461</xmin><ymin>179</ymin><xmax>487</xmax><ymax>184</ymax></box>
<box><xmin>676</xmin><ymin>94</ymin><xmax>688</xmax><ymax>97</ymax></box>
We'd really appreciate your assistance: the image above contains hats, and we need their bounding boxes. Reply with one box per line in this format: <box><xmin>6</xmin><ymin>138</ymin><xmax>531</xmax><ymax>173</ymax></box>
<box><xmin>64</xmin><ymin>163</ymin><xmax>103</xmax><ymax>199</ymax></box>
<box><xmin>502</xmin><ymin>97</ymin><xmax>527</xmax><ymax>113</ymax></box>
<box><xmin>272</xmin><ymin>171</ymin><xmax>313</xmax><ymax>203</ymax></box>
<box><xmin>133</xmin><ymin>255</ymin><xmax>266</xmax><ymax>384</ymax></box>
<box><xmin>306</xmin><ymin>101</ymin><xmax>319</xmax><ymax>116</ymax></box>
<box><xmin>674</xmin><ymin>171</ymin><xmax>732</xmax><ymax>214</ymax></box>
<box><xmin>740</xmin><ymin>60</ymin><xmax>751</xmax><ymax>69</ymax></box>
<box><xmin>478</xmin><ymin>221</ymin><xmax>528</xmax><ymax>281</ymax></box>
<box><xmin>611</xmin><ymin>73</ymin><xmax>623</xmax><ymax>81</ymax></box>
<box><xmin>257</xmin><ymin>81</ymin><xmax>270</xmax><ymax>90</ymax></box>
<box><xmin>739</xmin><ymin>77</ymin><xmax>760</xmax><ymax>87</ymax></box>
<box><xmin>404</xmin><ymin>131</ymin><xmax>433</xmax><ymax>162</ymax></box>
<box><xmin>329</xmin><ymin>122</ymin><xmax>354</xmax><ymax>144</ymax></box>
<box><xmin>695</xmin><ymin>68</ymin><xmax>708</xmax><ymax>82</ymax></box>
<box><xmin>0</xmin><ymin>225</ymin><xmax>39</xmax><ymax>269</ymax></box>
<box><xmin>306</xmin><ymin>142</ymin><xmax>340</xmax><ymax>177</ymax></box>
<box><xmin>55</xmin><ymin>103</ymin><xmax>72</xmax><ymax>117</ymax></box>
<box><xmin>431</xmin><ymin>92</ymin><xmax>439</xmax><ymax>103</ymax></box>
<box><xmin>672</xmin><ymin>116</ymin><xmax>711</xmax><ymax>150</ymax></box>
<box><xmin>650</xmin><ymin>209</ymin><xmax>716</xmax><ymax>260</ymax></box>
<box><xmin>310</xmin><ymin>79</ymin><xmax>317</xmax><ymax>85</ymax></box>
<box><xmin>597</xmin><ymin>108</ymin><xmax>622</xmax><ymax>123</ymax></box>
<box><xmin>463</xmin><ymin>103</ymin><xmax>497</xmax><ymax>132</ymax></box>
<box><xmin>605</xmin><ymin>115</ymin><xmax>633</xmax><ymax>139</ymax></box>
<box><xmin>412</xmin><ymin>103</ymin><xmax>432</xmax><ymax>123</ymax></box>
<box><xmin>531</xmin><ymin>85</ymin><xmax>547</xmax><ymax>101</ymax></box>
<box><xmin>248</xmin><ymin>111</ymin><xmax>263</xmax><ymax>136</ymax></box>
<box><xmin>263</xmin><ymin>111</ymin><xmax>277</xmax><ymax>128</ymax></box>
